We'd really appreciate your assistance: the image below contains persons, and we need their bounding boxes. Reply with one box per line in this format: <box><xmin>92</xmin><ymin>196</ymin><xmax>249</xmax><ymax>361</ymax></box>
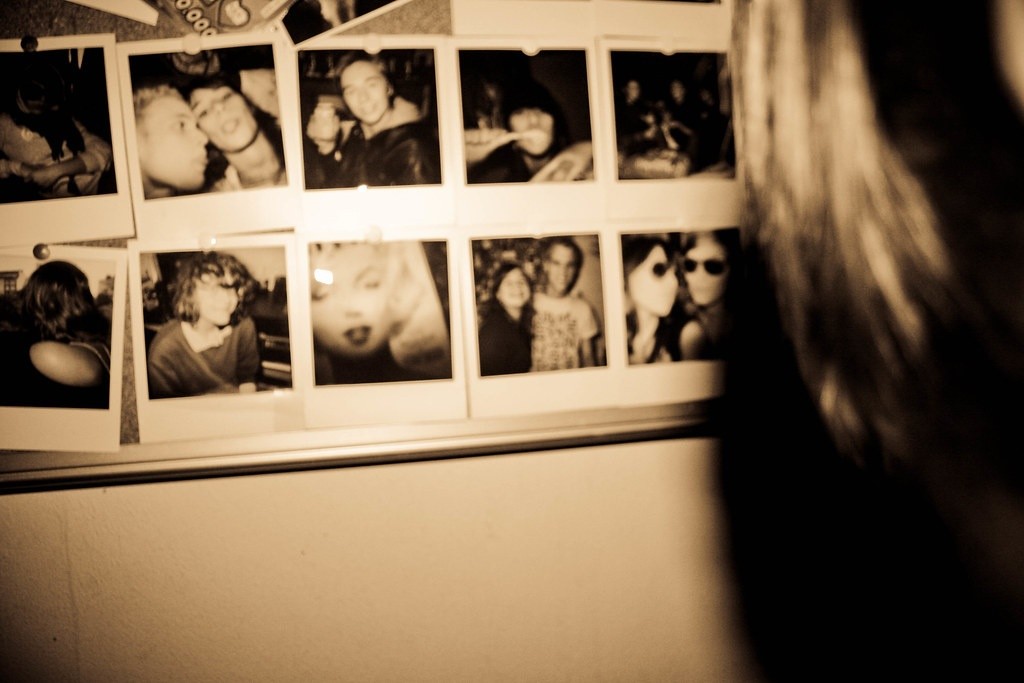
<box><xmin>728</xmin><ymin>0</ymin><xmax>1024</xmax><ymax>587</ymax></box>
<box><xmin>0</xmin><ymin>51</ymin><xmax>735</xmax><ymax>409</ymax></box>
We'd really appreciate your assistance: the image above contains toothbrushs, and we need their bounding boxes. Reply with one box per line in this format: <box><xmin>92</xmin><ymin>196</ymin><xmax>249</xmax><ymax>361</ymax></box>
<box><xmin>491</xmin><ymin>131</ymin><xmax>544</xmax><ymax>150</ymax></box>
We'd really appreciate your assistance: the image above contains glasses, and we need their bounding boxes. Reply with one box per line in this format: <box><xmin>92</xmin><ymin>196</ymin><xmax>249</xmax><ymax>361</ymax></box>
<box><xmin>684</xmin><ymin>258</ymin><xmax>726</xmax><ymax>275</ymax></box>
<box><xmin>653</xmin><ymin>262</ymin><xmax>666</xmax><ymax>276</ymax></box>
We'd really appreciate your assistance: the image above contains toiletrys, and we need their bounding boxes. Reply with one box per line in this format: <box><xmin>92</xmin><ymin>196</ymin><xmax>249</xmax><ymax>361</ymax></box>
<box><xmin>529</xmin><ymin>142</ymin><xmax>592</xmax><ymax>182</ymax></box>
